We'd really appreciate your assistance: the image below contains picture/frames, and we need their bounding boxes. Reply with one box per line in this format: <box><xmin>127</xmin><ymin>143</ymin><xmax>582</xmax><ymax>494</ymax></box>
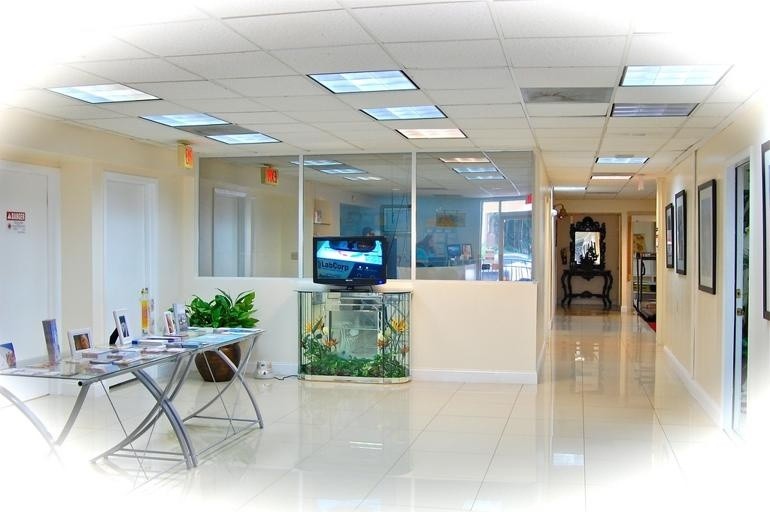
<box><xmin>68</xmin><ymin>332</ymin><xmax>93</xmax><ymax>354</ymax></box>
<box><xmin>675</xmin><ymin>191</ymin><xmax>686</xmax><ymax>274</ymax></box>
<box><xmin>665</xmin><ymin>203</ymin><xmax>674</xmax><ymax>269</ymax></box>
<box><xmin>762</xmin><ymin>139</ymin><xmax>770</xmax><ymax>319</ymax></box>
<box><xmin>110</xmin><ymin>309</ymin><xmax>132</xmax><ymax>344</ymax></box>
<box><xmin>698</xmin><ymin>180</ymin><xmax>716</xmax><ymax>294</ymax></box>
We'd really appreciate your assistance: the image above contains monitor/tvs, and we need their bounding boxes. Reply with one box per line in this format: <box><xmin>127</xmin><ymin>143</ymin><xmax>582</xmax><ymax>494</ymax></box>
<box><xmin>312</xmin><ymin>236</ymin><xmax>388</xmax><ymax>293</ymax></box>
<box><xmin>385</xmin><ymin>239</ymin><xmax>398</xmax><ymax>279</ymax></box>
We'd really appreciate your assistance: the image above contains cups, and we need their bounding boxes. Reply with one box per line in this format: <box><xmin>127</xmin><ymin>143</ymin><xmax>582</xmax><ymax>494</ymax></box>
<box><xmin>178</xmin><ymin>314</ymin><xmax>187</xmax><ymax>330</ymax></box>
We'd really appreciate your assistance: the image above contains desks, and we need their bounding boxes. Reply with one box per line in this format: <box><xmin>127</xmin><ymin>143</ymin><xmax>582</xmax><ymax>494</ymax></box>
<box><xmin>561</xmin><ymin>269</ymin><xmax>613</xmax><ymax>310</ymax></box>
<box><xmin>0</xmin><ymin>327</ymin><xmax>267</xmax><ymax>483</ymax></box>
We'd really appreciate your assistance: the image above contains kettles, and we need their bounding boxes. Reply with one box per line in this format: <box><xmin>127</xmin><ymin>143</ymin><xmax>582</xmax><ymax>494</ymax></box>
<box><xmin>255</xmin><ymin>360</ymin><xmax>273</xmax><ymax>378</ymax></box>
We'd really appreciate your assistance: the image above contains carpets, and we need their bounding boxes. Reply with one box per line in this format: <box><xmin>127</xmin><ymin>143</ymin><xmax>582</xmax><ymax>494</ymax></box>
<box><xmin>633</xmin><ymin>294</ymin><xmax>656</xmax><ymax>332</ymax></box>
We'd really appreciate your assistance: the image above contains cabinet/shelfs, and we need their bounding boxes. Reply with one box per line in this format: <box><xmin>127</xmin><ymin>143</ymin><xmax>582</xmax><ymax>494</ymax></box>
<box><xmin>634</xmin><ymin>284</ymin><xmax>657</xmax><ymax>292</ymax></box>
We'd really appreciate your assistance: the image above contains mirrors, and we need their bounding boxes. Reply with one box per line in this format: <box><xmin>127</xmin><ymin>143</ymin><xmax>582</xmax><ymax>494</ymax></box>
<box><xmin>569</xmin><ymin>217</ymin><xmax>606</xmax><ymax>270</ymax></box>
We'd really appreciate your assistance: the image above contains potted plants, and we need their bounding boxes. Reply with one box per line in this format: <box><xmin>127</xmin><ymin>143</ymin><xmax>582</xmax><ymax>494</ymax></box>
<box><xmin>184</xmin><ymin>288</ymin><xmax>259</xmax><ymax>384</ymax></box>
